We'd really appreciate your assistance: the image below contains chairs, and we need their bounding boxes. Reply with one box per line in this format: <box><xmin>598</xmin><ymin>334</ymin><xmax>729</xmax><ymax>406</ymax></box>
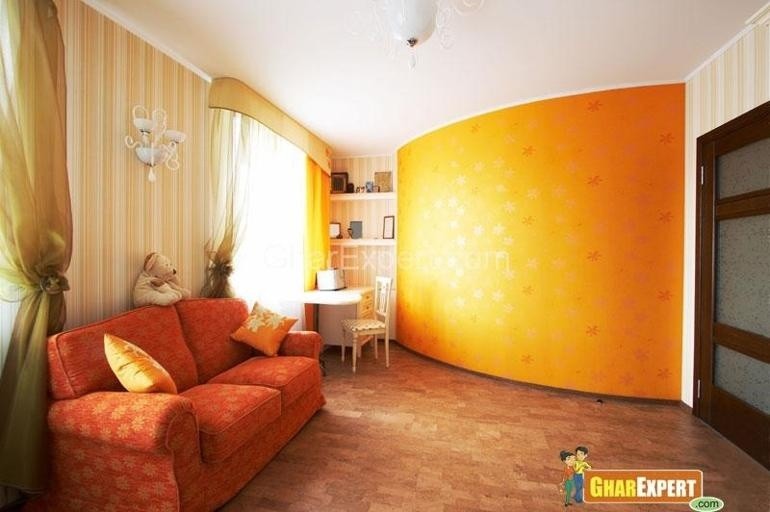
<box><xmin>339</xmin><ymin>278</ymin><xmax>390</xmax><ymax>374</ymax></box>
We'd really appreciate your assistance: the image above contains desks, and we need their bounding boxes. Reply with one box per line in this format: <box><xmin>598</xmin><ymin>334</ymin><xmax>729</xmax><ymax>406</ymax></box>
<box><xmin>302</xmin><ymin>285</ymin><xmax>376</xmax><ymax>358</ymax></box>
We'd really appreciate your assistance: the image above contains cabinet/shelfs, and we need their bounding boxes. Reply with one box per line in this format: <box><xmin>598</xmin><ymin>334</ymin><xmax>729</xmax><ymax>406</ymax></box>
<box><xmin>329</xmin><ymin>193</ymin><xmax>397</xmax><ymax>247</ymax></box>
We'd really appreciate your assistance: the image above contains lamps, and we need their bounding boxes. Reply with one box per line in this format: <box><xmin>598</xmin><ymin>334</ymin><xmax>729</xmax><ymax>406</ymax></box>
<box><xmin>377</xmin><ymin>0</ymin><xmax>485</xmax><ymax>49</ymax></box>
<box><xmin>125</xmin><ymin>106</ymin><xmax>187</xmax><ymax>182</ymax></box>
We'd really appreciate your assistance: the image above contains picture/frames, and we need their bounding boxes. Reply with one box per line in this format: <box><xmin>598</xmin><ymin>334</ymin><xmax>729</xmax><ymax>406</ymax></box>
<box><xmin>383</xmin><ymin>216</ymin><xmax>394</xmax><ymax>238</ymax></box>
<box><xmin>332</xmin><ymin>172</ymin><xmax>348</xmax><ymax>193</ymax></box>
<box><xmin>329</xmin><ymin>223</ymin><xmax>341</xmax><ymax>238</ymax></box>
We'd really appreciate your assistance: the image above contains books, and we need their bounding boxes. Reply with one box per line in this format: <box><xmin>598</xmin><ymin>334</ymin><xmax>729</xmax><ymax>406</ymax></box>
<box><xmin>375</xmin><ymin>171</ymin><xmax>393</xmax><ymax>193</ymax></box>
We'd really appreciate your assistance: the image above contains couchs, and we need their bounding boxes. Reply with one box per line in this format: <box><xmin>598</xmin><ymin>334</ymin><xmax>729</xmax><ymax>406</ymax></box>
<box><xmin>35</xmin><ymin>298</ymin><xmax>327</xmax><ymax>512</ymax></box>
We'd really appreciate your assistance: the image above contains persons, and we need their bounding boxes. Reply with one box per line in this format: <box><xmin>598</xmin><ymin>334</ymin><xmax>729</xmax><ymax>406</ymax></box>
<box><xmin>559</xmin><ymin>450</ymin><xmax>575</xmax><ymax>506</ymax></box>
<box><xmin>133</xmin><ymin>251</ymin><xmax>192</xmax><ymax>306</ymax></box>
<box><xmin>572</xmin><ymin>446</ymin><xmax>592</xmax><ymax>502</ymax></box>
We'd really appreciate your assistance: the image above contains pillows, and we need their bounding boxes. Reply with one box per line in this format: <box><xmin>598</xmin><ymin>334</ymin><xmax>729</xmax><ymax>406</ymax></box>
<box><xmin>230</xmin><ymin>301</ymin><xmax>299</xmax><ymax>356</ymax></box>
<box><xmin>104</xmin><ymin>332</ymin><xmax>178</xmax><ymax>395</ymax></box>
